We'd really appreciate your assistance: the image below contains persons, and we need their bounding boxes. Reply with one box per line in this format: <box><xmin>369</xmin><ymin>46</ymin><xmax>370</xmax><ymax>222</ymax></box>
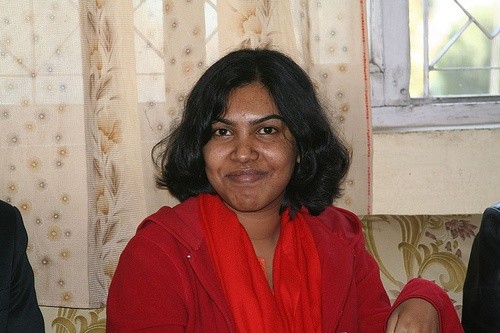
<box><xmin>106</xmin><ymin>48</ymin><xmax>464</xmax><ymax>333</ymax></box>
<box><xmin>0</xmin><ymin>200</ymin><xmax>45</xmax><ymax>333</ymax></box>
<box><xmin>461</xmin><ymin>202</ymin><xmax>500</xmax><ymax>333</ymax></box>
